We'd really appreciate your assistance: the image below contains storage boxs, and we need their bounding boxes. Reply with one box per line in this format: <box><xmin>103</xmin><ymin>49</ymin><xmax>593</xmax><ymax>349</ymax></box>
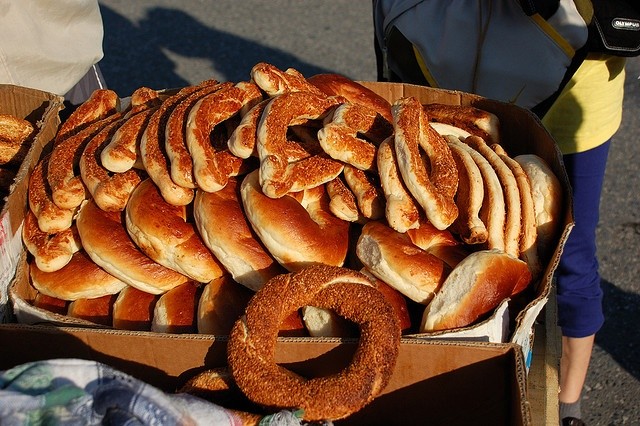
<box><xmin>1</xmin><ymin>83</ymin><xmax>67</xmax><ymax>322</ymax></box>
<box><xmin>1</xmin><ymin>322</ymin><xmax>535</xmax><ymax>426</ymax></box>
<box><xmin>8</xmin><ymin>80</ymin><xmax>577</xmax><ymax>374</ymax></box>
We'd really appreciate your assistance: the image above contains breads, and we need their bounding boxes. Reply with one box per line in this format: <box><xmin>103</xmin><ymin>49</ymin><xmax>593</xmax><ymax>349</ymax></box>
<box><xmin>1</xmin><ymin>113</ymin><xmax>34</xmax><ymax>203</ymax></box>
<box><xmin>17</xmin><ymin>63</ymin><xmax>564</xmax><ymax>421</ymax></box>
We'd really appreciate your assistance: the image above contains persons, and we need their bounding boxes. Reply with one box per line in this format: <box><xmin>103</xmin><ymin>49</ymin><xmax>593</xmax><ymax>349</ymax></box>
<box><xmin>372</xmin><ymin>0</ymin><xmax>640</xmax><ymax>426</ymax></box>
<box><xmin>0</xmin><ymin>0</ymin><xmax>107</xmax><ymax>124</ymax></box>
